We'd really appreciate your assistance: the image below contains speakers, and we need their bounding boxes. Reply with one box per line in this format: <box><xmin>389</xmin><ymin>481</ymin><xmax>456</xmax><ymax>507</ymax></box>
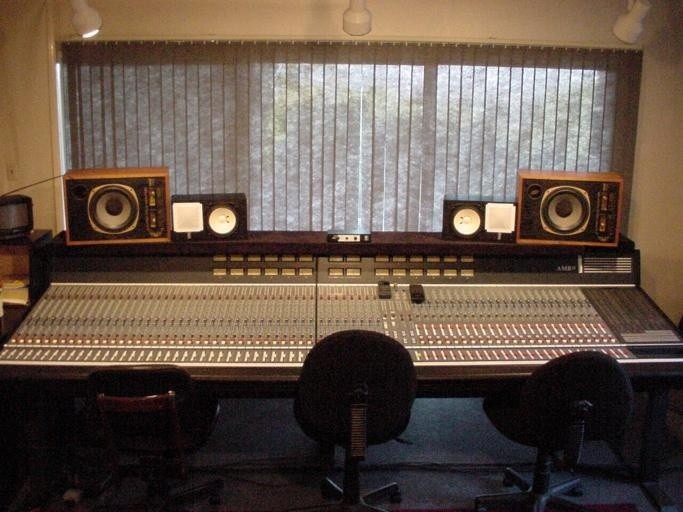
<box><xmin>171</xmin><ymin>193</ymin><xmax>247</xmax><ymax>242</ymax></box>
<box><xmin>443</xmin><ymin>192</ymin><xmax>516</xmax><ymax>244</ymax></box>
<box><xmin>63</xmin><ymin>167</ymin><xmax>172</xmax><ymax>245</ymax></box>
<box><xmin>516</xmin><ymin>168</ymin><xmax>624</xmax><ymax>246</ymax></box>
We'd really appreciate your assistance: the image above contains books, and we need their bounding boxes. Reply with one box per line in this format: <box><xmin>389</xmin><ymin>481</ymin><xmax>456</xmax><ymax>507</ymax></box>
<box><xmin>0</xmin><ymin>272</ymin><xmax>29</xmax><ymax>290</ymax></box>
<box><xmin>0</xmin><ymin>288</ymin><xmax>28</xmax><ymax>306</ymax></box>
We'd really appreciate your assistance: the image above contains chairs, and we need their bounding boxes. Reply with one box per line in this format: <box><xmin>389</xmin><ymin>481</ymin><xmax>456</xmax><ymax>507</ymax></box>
<box><xmin>71</xmin><ymin>362</ymin><xmax>224</xmax><ymax>512</ymax></box>
<box><xmin>473</xmin><ymin>349</ymin><xmax>636</xmax><ymax>512</ymax></box>
<box><xmin>290</xmin><ymin>330</ymin><xmax>418</xmax><ymax>511</ymax></box>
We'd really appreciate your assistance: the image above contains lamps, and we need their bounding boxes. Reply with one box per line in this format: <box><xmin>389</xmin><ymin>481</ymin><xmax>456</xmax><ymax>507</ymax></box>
<box><xmin>611</xmin><ymin>1</ymin><xmax>653</xmax><ymax>45</ymax></box>
<box><xmin>65</xmin><ymin>0</ymin><xmax>102</xmax><ymax>40</ymax></box>
<box><xmin>341</xmin><ymin>0</ymin><xmax>373</xmax><ymax>36</ymax></box>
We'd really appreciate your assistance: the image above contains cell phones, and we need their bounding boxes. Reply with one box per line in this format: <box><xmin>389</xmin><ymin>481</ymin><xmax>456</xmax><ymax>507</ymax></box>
<box><xmin>410</xmin><ymin>284</ymin><xmax>425</xmax><ymax>303</ymax></box>
<box><xmin>378</xmin><ymin>280</ymin><xmax>392</xmax><ymax>298</ymax></box>
